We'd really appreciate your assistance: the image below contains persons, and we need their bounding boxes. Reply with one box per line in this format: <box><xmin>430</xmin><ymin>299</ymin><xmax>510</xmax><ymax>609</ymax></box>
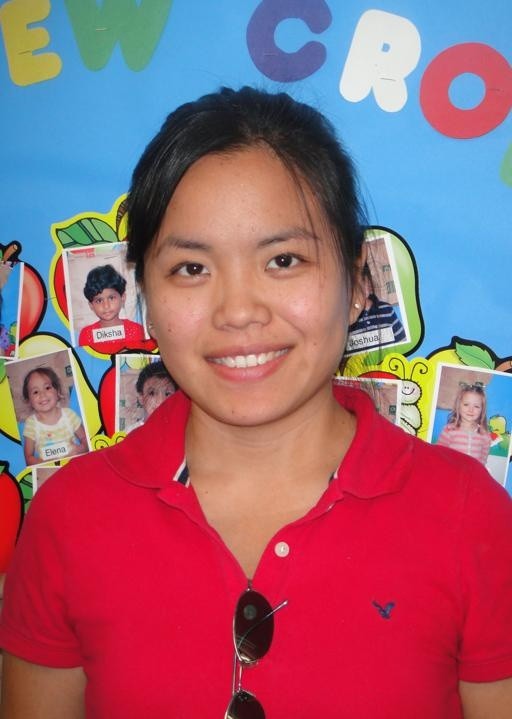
<box><xmin>125</xmin><ymin>361</ymin><xmax>177</xmax><ymax>429</ymax></box>
<box><xmin>20</xmin><ymin>365</ymin><xmax>92</xmax><ymax>466</ymax></box>
<box><xmin>342</xmin><ymin>261</ymin><xmax>406</xmax><ymax>355</ymax></box>
<box><xmin>2</xmin><ymin>81</ymin><xmax>510</xmax><ymax>719</ymax></box>
<box><xmin>78</xmin><ymin>264</ymin><xmax>144</xmax><ymax>351</ymax></box>
<box><xmin>435</xmin><ymin>380</ymin><xmax>490</xmax><ymax>471</ymax></box>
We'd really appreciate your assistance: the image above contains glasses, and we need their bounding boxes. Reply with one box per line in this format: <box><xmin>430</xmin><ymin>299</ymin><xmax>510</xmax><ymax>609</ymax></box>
<box><xmin>223</xmin><ymin>578</ymin><xmax>289</xmax><ymax>719</ymax></box>
